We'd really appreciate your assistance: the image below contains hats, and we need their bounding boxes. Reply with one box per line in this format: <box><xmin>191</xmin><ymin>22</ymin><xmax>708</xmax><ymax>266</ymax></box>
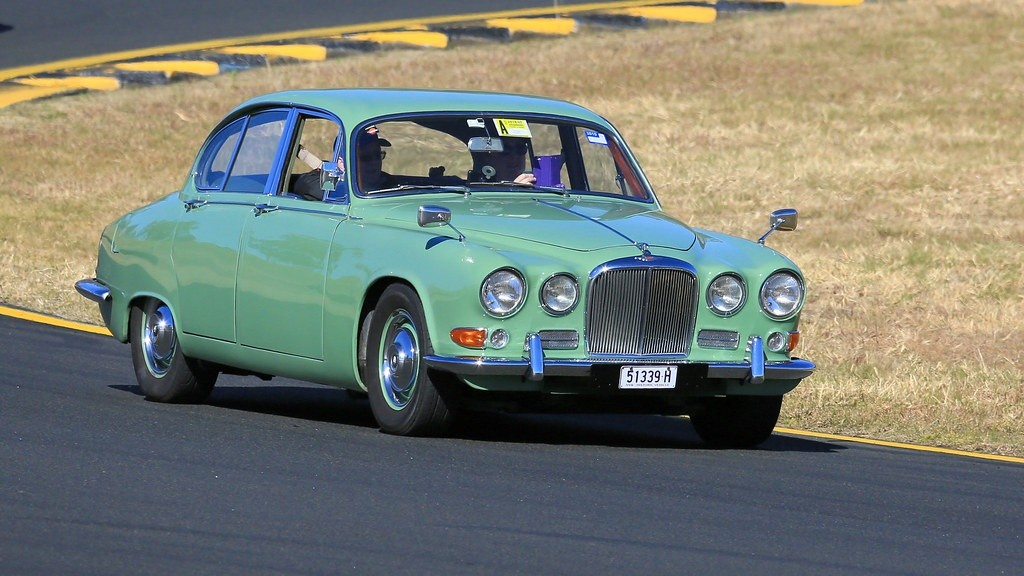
<box><xmin>333</xmin><ymin>127</ymin><xmax>392</xmax><ymax>151</ymax></box>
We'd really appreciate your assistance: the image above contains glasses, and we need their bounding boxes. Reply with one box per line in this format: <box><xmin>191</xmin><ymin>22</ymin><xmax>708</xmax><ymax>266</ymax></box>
<box><xmin>359</xmin><ymin>151</ymin><xmax>386</xmax><ymax>162</ymax></box>
<box><xmin>500</xmin><ymin>144</ymin><xmax>529</xmax><ymax>156</ymax></box>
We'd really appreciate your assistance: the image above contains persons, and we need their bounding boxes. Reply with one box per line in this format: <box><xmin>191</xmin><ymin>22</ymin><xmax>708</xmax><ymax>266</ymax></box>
<box><xmin>294</xmin><ymin>126</ymin><xmax>398</xmax><ymax>202</ymax></box>
<box><xmin>467</xmin><ymin>135</ymin><xmax>536</xmax><ymax>189</ymax></box>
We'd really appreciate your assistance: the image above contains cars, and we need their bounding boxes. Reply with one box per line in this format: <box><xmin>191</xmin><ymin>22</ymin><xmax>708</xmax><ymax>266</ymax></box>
<box><xmin>74</xmin><ymin>88</ymin><xmax>815</xmax><ymax>451</ymax></box>
